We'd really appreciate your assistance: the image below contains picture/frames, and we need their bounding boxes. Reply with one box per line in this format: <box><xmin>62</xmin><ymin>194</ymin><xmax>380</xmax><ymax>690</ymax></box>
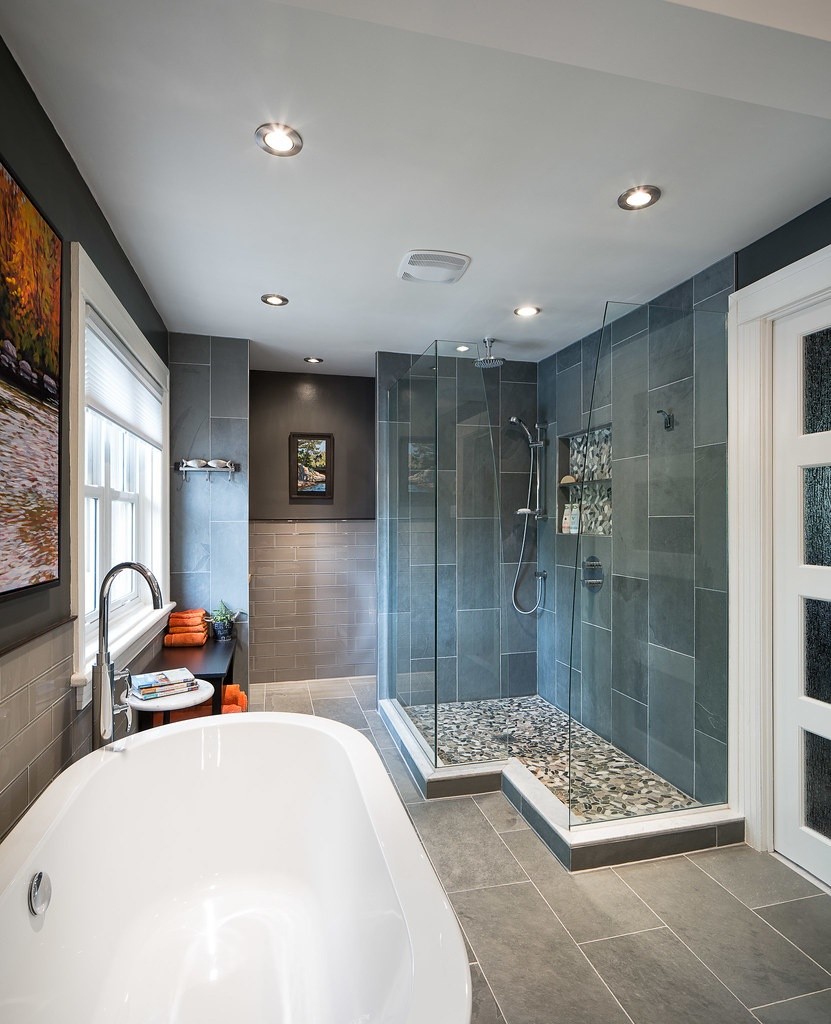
<box><xmin>0</xmin><ymin>154</ymin><xmax>64</xmax><ymax>602</ymax></box>
<box><xmin>401</xmin><ymin>437</ymin><xmax>439</xmax><ymax>506</ymax></box>
<box><xmin>290</xmin><ymin>432</ymin><xmax>334</xmax><ymax>500</ymax></box>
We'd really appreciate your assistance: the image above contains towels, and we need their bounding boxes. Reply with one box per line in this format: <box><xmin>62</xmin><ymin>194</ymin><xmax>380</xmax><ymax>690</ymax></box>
<box><xmin>164</xmin><ymin>607</ymin><xmax>208</xmax><ymax>647</ymax></box>
<box><xmin>152</xmin><ymin>683</ymin><xmax>248</xmax><ymax>727</ymax></box>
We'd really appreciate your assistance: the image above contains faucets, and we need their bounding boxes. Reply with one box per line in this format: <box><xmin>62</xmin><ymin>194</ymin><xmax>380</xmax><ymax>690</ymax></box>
<box><xmin>95</xmin><ymin>560</ymin><xmax>163</xmax><ymax>665</ymax></box>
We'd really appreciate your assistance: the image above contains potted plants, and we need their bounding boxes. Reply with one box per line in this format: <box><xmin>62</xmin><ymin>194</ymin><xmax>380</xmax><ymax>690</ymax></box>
<box><xmin>203</xmin><ymin>599</ymin><xmax>247</xmax><ymax>642</ymax></box>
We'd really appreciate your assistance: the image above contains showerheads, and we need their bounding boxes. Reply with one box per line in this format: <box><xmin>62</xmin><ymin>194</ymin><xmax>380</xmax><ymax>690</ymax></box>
<box><xmin>473</xmin><ymin>356</ymin><xmax>506</xmax><ymax>369</ymax></box>
<box><xmin>508</xmin><ymin>415</ymin><xmax>536</xmax><ymax>441</ymax></box>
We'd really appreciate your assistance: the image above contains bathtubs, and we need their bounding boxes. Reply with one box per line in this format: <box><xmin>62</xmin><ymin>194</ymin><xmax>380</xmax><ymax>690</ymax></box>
<box><xmin>0</xmin><ymin>709</ymin><xmax>474</xmax><ymax>1024</ymax></box>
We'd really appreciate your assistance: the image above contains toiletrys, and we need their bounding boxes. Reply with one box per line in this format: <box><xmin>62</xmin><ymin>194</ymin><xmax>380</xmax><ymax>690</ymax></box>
<box><xmin>561</xmin><ymin>503</ymin><xmax>582</xmax><ymax>534</ymax></box>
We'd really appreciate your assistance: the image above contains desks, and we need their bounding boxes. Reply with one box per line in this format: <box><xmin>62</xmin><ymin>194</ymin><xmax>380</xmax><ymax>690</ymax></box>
<box><xmin>137</xmin><ymin>636</ymin><xmax>237</xmax><ymax>732</ymax></box>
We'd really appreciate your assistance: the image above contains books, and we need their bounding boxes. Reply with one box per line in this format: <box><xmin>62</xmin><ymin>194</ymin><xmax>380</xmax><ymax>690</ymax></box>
<box><xmin>129</xmin><ymin>665</ymin><xmax>198</xmax><ymax>701</ymax></box>
